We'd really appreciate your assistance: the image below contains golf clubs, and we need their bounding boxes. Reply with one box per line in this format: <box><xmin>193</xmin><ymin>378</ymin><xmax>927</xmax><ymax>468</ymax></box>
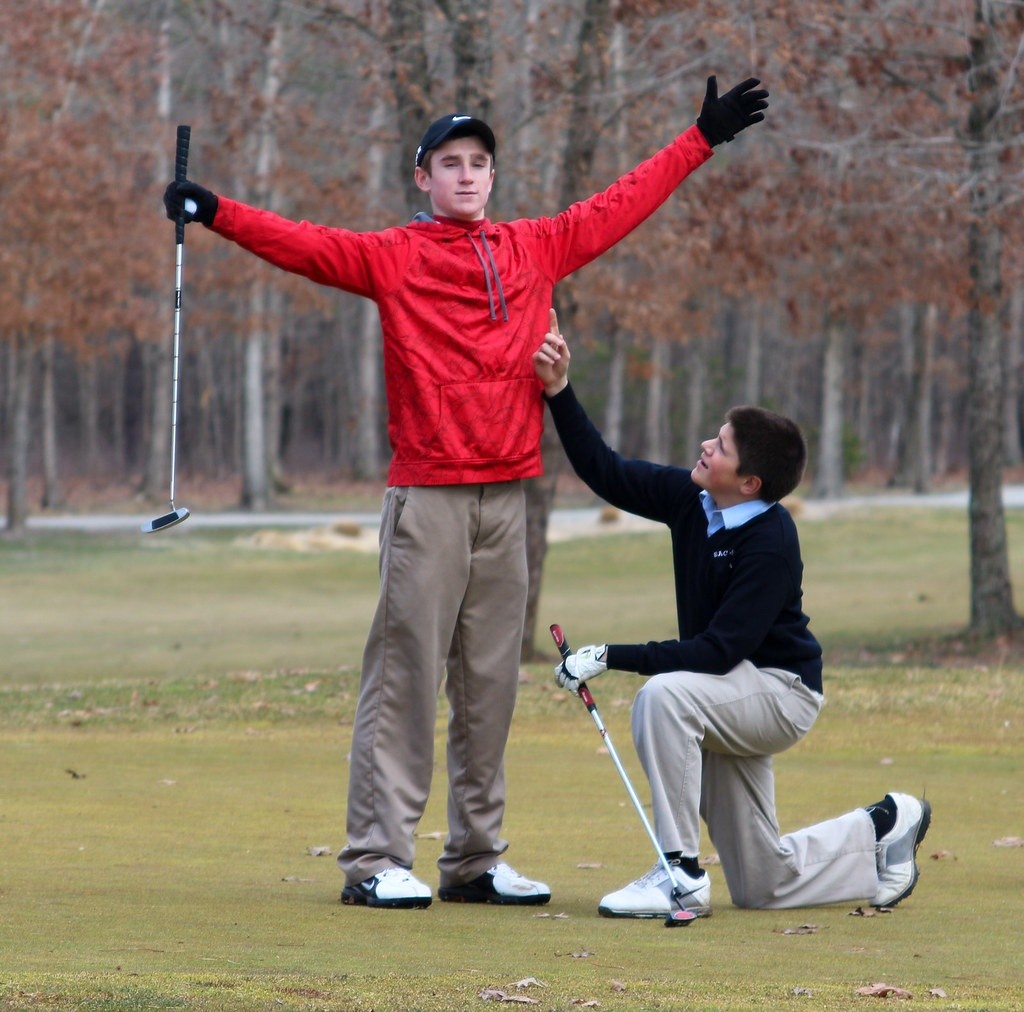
<box><xmin>548</xmin><ymin>621</ymin><xmax>698</xmax><ymax>928</ymax></box>
<box><xmin>139</xmin><ymin>124</ymin><xmax>194</xmax><ymax>538</ymax></box>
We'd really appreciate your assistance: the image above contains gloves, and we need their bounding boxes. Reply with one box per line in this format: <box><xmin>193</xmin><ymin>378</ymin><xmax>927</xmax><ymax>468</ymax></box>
<box><xmin>164</xmin><ymin>179</ymin><xmax>218</xmax><ymax>227</ymax></box>
<box><xmin>696</xmin><ymin>75</ymin><xmax>769</xmax><ymax>149</ymax></box>
<box><xmin>554</xmin><ymin>642</ymin><xmax>608</xmax><ymax>698</ymax></box>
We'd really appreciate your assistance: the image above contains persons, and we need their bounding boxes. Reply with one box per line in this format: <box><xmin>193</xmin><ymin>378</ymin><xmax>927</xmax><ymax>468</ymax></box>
<box><xmin>534</xmin><ymin>308</ymin><xmax>932</xmax><ymax>919</ymax></box>
<box><xmin>163</xmin><ymin>73</ymin><xmax>768</xmax><ymax>908</ymax></box>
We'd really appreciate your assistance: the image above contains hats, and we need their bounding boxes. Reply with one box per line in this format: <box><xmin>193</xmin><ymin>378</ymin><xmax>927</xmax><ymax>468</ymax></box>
<box><xmin>414</xmin><ymin>114</ymin><xmax>496</xmax><ymax>166</ymax></box>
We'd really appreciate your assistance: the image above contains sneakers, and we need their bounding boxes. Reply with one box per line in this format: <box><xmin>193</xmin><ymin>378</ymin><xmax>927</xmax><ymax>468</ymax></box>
<box><xmin>437</xmin><ymin>863</ymin><xmax>551</xmax><ymax>905</ymax></box>
<box><xmin>342</xmin><ymin>867</ymin><xmax>433</xmax><ymax>908</ymax></box>
<box><xmin>598</xmin><ymin>857</ymin><xmax>711</xmax><ymax>919</ymax></box>
<box><xmin>869</xmin><ymin>792</ymin><xmax>931</xmax><ymax>907</ymax></box>
